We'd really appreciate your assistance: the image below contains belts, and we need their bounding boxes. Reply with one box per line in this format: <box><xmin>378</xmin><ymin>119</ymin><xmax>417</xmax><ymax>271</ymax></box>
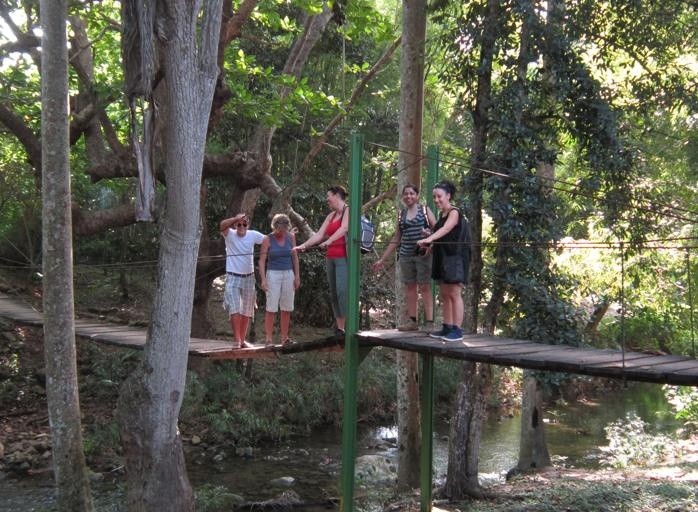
<box><xmin>229</xmin><ymin>272</ymin><xmax>250</xmax><ymax>277</ymax></box>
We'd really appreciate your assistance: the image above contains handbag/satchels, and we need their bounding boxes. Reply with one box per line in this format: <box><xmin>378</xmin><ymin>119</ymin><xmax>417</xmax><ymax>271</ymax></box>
<box><xmin>360</xmin><ymin>216</ymin><xmax>375</xmax><ymax>254</ymax></box>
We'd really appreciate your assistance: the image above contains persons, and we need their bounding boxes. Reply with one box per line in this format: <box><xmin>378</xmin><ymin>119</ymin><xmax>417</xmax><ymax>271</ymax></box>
<box><xmin>291</xmin><ymin>184</ymin><xmax>350</xmax><ymax>336</ymax></box>
<box><xmin>220</xmin><ymin>212</ymin><xmax>267</xmax><ymax>349</ymax></box>
<box><xmin>373</xmin><ymin>184</ymin><xmax>437</xmax><ymax>332</ymax></box>
<box><xmin>259</xmin><ymin>214</ymin><xmax>300</xmax><ymax>347</ymax></box>
<box><xmin>416</xmin><ymin>180</ymin><xmax>472</xmax><ymax>341</ymax></box>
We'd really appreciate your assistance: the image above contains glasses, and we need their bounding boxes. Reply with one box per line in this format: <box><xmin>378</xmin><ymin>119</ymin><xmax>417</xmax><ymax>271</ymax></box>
<box><xmin>237</xmin><ymin>223</ymin><xmax>248</xmax><ymax>228</ymax></box>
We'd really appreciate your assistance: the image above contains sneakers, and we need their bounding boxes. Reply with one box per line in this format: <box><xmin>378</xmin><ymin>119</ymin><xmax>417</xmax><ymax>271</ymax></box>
<box><xmin>416</xmin><ymin>321</ymin><xmax>435</xmax><ymax>337</ymax></box>
<box><xmin>281</xmin><ymin>338</ymin><xmax>294</xmax><ymax>346</ymax></box>
<box><xmin>428</xmin><ymin>324</ymin><xmax>463</xmax><ymax>341</ymax></box>
<box><xmin>398</xmin><ymin>319</ymin><xmax>418</xmax><ymax>331</ymax></box>
<box><xmin>232</xmin><ymin>341</ymin><xmax>250</xmax><ymax>350</ymax></box>
<box><xmin>265</xmin><ymin>341</ymin><xmax>275</xmax><ymax>348</ymax></box>
